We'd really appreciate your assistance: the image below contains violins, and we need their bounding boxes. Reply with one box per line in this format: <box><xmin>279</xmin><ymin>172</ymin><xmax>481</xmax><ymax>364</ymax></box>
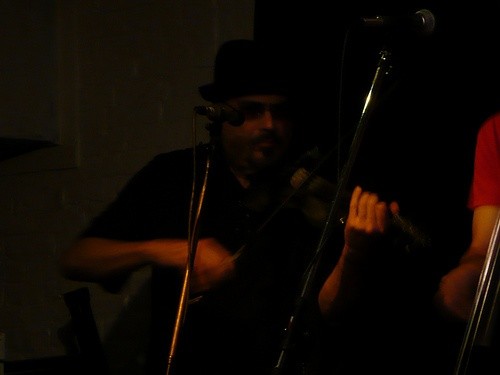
<box><xmin>276</xmin><ymin>149</ymin><xmax>429</xmax><ymax>248</ymax></box>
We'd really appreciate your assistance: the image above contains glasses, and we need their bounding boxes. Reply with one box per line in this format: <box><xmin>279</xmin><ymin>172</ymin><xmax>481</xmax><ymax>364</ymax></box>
<box><xmin>229</xmin><ymin>100</ymin><xmax>291</xmax><ymax>119</ymax></box>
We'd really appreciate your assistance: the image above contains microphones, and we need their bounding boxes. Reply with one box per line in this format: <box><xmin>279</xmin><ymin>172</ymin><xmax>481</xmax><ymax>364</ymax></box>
<box><xmin>195</xmin><ymin>104</ymin><xmax>245</xmax><ymax>127</ymax></box>
<box><xmin>362</xmin><ymin>10</ymin><xmax>435</xmax><ymax>32</ymax></box>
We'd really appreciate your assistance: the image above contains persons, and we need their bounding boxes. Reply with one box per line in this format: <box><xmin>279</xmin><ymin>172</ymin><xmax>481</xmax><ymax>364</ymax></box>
<box><xmin>58</xmin><ymin>40</ymin><xmax>401</xmax><ymax>375</ymax></box>
<box><xmin>437</xmin><ymin>113</ymin><xmax>500</xmax><ymax>322</ymax></box>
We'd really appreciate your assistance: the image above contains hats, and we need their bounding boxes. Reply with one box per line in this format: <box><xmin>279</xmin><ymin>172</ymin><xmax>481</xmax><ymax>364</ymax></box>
<box><xmin>197</xmin><ymin>38</ymin><xmax>295</xmax><ymax>104</ymax></box>
